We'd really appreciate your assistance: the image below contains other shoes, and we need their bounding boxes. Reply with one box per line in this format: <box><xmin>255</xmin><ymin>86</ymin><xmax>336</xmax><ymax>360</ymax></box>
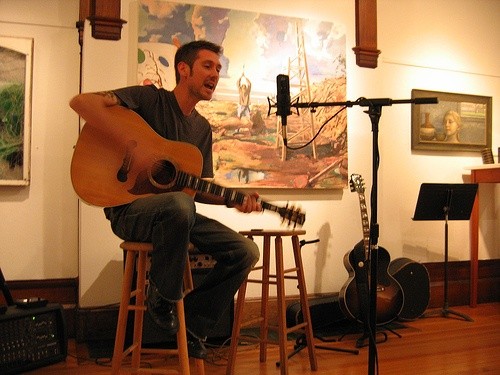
<box><xmin>145</xmin><ymin>276</ymin><xmax>180</xmax><ymax>338</ymax></box>
<box><xmin>178</xmin><ymin>327</ymin><xmax>208</xmax><ymax>359</ymax></box>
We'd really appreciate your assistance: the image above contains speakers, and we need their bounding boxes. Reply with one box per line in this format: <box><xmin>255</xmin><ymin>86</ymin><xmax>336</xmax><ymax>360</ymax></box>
<box><xmin>0</xmin><ymin>303</ymin><xmax>68</xmax><ymax>375</ymax></box>
<box><xmin>125</xmin><ymin>260</ymin><xmax>235</xmax><ymax>346</ymax></box>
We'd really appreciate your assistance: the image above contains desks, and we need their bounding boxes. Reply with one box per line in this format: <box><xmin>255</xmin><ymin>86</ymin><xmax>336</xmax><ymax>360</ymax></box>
<box><xmin>466</xmin><ymin>164</ymin><xmax>500</xmax><ymax>308</ymax></box>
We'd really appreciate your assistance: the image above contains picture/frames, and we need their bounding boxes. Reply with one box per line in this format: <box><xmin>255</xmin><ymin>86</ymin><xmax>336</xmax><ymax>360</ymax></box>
<box><xmin>411</xmin><ymin>89</ymin><xmax>492</xmax><ymax>151</ymax></box>
<box><xmin>0</xmin><ymin>36</ymin><xmax>34</xmax><ymax>187</ymax></box>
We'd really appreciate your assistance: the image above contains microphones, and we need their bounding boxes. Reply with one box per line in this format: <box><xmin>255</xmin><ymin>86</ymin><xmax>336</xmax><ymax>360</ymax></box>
<box><xmin>277</xmin><ymin>73</ymin><xmax>291</xmax><ymax>141</ymax></box>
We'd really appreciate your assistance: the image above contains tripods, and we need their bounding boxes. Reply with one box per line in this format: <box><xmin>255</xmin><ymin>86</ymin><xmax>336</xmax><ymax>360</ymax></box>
<box><xmin>410</xmin><ymin>183</ymin><xmax>478</xmax><ymax>323</ymax></box>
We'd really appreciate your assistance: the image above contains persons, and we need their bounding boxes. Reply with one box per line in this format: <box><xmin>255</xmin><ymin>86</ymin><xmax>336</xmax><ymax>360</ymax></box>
<box><xmin>69</xmin><ymin>39</ymin><xmax>260</xmax><ymax>359</ymax></box>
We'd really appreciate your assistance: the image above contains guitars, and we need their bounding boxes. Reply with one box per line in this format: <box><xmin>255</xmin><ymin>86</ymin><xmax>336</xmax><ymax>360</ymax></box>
<box><xmin>338</xmin><ymin>174</ymin><xmax>406</xmax><ymax>327</ymax></box>
<box><xmin>71</xmin><ymin>105</ymin><xmax>307</xmax><ymax>229</ymax></box>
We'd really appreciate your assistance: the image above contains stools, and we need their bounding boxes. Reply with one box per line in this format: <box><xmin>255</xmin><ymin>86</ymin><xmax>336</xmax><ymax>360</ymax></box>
<box><xmin>111</xmin><ymin>241</ymin><xmax>205</xmax><ymax>375</ymax></box>
<box><xmin>226</xmin><ymin>231</ymin><xmax>317</xmax><ymax>375</ymax></box>
